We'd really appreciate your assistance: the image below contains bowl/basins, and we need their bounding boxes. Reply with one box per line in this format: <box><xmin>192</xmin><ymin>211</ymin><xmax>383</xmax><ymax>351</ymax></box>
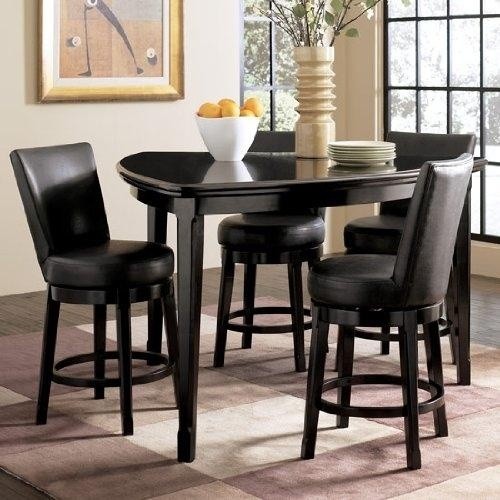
<box><xmin>194</xmin><ymin>112</ymin><xmax>261</xmax><ymax>162</ymax></box>
<box><xmin>200</xmin><ymin>161</ymin><xmax>254</xmax><ymax>184</ymax></box>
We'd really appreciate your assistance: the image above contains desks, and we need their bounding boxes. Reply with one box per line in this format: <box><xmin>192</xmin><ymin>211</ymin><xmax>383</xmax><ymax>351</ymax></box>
<box><xmin>116</xmin><ymin>150</ymin><xmax>489</xmax><ymax>463</ymax></box>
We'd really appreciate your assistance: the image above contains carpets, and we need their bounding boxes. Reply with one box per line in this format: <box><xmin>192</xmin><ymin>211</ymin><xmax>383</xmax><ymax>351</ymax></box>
<box><xmin>1</xmin><ymin>254</ymin><xmax>499</xmax><ymax>495</ymax></box>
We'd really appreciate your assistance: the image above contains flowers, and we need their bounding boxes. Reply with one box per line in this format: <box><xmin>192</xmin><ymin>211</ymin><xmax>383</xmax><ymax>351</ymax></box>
<box><xmin>241</xmin><ymin>0</ymin><xmax>411</xmax><ymax>47</ymax></box>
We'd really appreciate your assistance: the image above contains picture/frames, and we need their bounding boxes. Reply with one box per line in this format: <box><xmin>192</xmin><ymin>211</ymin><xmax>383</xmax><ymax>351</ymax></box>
<box><xmin>36</xmin><ymin>0</ymin><xmax>185</xmax><ymax>104</ymax></box>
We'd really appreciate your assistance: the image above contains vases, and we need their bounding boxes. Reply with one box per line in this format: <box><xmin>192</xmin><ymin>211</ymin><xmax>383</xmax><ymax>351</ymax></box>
<box><xmin>291</xmin><ymin>46</ymin><xmax>337</xmax><ymax>159</ymax></box>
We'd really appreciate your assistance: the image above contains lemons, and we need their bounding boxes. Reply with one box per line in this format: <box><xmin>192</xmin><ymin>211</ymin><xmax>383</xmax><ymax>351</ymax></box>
<box><xmin>198</xmin><ymin>102</ymin><xmax>218</xmax><ymax>118</ymax></box>
<box><xmin>244</xmin><ymin>97</ymin><xmax>263</xmax><ymax>117</ymax></box>
<box><xmin>240</xmin><ymin>109</ymin><xmax>254</xmax><ymax>117</ymax></box>
<box><xmin>216</xmin><ymin>107</ymin><xmax>223</xmax><ymax>118</ymax></box>
<box><xmin>222</xmin><ymin>103</ymin><xmax>238</xmax><ymax>118</ymax></box>
<box><xmin>217</xmin><ymin>98</ymin><xmax>236</xmax><ymax>106</ymax></box>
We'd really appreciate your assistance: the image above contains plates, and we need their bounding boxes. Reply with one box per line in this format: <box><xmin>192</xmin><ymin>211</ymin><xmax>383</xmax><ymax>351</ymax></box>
<box><xmin>326</xmin><ymin>165</ymin><xmax>398</xmax><ymax>179</ymax></box>
<box><xmin>326</xmin><ymin>141</ymin><xmax>397</xmax><ymax>168</ymax></box>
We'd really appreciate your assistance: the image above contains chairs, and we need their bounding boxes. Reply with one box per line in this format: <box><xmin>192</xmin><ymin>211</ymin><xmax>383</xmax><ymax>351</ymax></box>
<box><xmin>334</xmin><ymin>130</ymin><xmax>477</xmax><ymax>385</ymax></box>
<box><xmin>213</xmin><ymin>130</ymin><xmax>329</xmax><ymax>373</ymax></box>
<box><xmin>300</xmin><ymin>153</ymin><xmax>474</xmax><ymax>471</ymax></box>
<box><xmin>9</xmin><ymin>142</ymin><xmax>179</xmax><ymax>436</ymax></box>
